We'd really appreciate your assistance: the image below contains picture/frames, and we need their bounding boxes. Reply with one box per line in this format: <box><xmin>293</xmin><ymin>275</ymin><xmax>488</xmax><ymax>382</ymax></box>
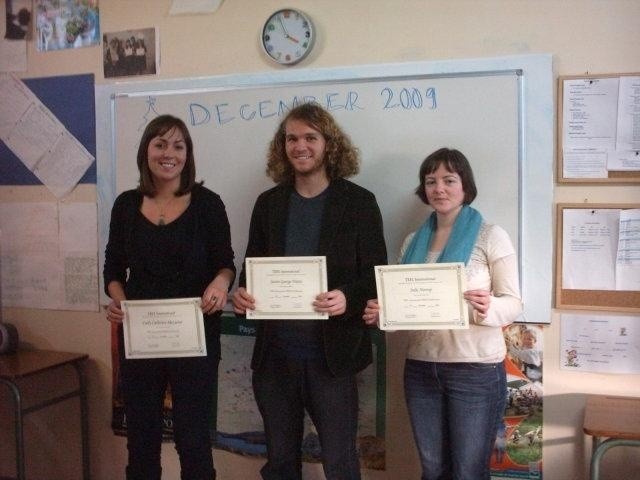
<box><xmin>103</xmin><ymin>27</ymin><xmax>160</xmax><ymax>79</ymax></box>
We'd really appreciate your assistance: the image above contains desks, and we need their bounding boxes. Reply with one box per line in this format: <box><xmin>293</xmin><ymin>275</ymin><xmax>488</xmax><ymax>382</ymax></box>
<box><xmin>582</xmin><ymin>395</ymin><xmax>640</xmax><ymax>480</ymax></box>
<box><xmin>0</xmin><ymin>349</ymin><xmax>91</xmax><ymax>480</ymax></box>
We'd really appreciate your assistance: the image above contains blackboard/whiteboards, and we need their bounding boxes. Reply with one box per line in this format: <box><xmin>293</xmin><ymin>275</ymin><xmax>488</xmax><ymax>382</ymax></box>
<box><xmin>111</xmin><ymin>67</ymin><xmax>524</xmax><ymax>314</ymax></box>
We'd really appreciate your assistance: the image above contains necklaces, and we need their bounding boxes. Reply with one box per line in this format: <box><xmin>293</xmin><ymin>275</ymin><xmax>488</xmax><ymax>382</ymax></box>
<box><xmin>155</xmin><ymin>192</ymin><xmax>173</xmax><ymax>226</ymax></box>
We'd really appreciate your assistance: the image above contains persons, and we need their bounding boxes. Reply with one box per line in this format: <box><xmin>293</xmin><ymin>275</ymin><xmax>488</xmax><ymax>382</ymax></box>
<box><xmin>231</xmin><ymin>102</ymin><xmax>387</xmax><ymax>480</ymax></box>
<box><xmin>103</xmin><ymin>114</ymin><xmax>237</xmax><ymax>480</ymax></box>
<box><xmin>364</xmin><ymin>147</ymin><xmax>523</xmax><ymax>479</ymax></box>
<box><xmin>103</xmin><ymin>36</ymin><xmax>147</xmax><ymax>76</ymax></box>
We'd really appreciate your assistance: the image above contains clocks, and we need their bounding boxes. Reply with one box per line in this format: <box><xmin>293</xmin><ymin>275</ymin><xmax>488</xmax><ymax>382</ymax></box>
<box><xmin>259</xmin><ymin>8</ymin><xmax>315</xmax><ymax>66</ymax></box>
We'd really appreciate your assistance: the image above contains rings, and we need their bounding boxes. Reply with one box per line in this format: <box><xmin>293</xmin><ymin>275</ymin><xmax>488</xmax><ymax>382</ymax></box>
<box><xmin>211</xmin><ymin>295</ymin><xmax>217</xmax><ymax>303</ymax></box>
